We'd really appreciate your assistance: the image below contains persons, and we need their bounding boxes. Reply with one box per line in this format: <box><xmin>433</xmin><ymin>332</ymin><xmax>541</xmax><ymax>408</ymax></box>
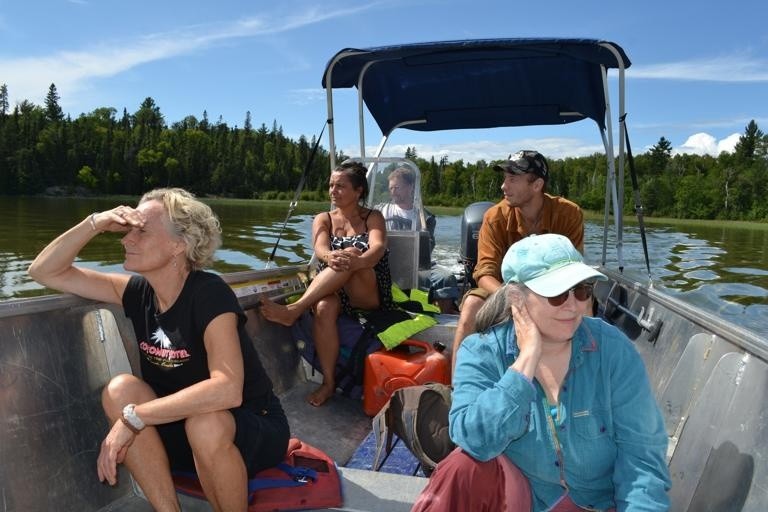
<box><xmin>410</xmin><ymin>234</ymin><xmax>672</xmax><ymax>512</ymax></box>
<box><xmin>260</xmin><ymin>162</ymin><xmax>392</xmax><ymax>406</ymax></box>
<box><xmin>452</xmin><ymin>150</ymin><xmax>593</xmax><ymax>377</ymax></box>
<box><xmin>374</xmin><ymin>168</ymin><xmax>458</xmax><ymax>314</ymax></box>
<box><xmin>28</xmin><ymin>187</ymin><xmax>290</xmax><ymax>512</ymax></box>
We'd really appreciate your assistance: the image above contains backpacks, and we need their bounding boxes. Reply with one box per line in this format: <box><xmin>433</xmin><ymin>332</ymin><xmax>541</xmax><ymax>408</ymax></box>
<box><xmin>373</xmin><ymin>382</ymin><xmax>457</xmax><ymax>479</ymax></box>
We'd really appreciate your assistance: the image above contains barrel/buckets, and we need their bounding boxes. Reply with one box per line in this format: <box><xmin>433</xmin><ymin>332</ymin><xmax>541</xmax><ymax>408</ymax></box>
<box><xmin>363</xmin><ymin>339</ymin><xmax>447</xmax><ymax>416</ymax></box>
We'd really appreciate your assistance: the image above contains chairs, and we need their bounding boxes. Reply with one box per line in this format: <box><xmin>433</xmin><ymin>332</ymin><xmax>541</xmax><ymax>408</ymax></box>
<box><xmin>306</xmin><ymin>231</ymin><xmax>425</xmax><ymax>406</ymax></box>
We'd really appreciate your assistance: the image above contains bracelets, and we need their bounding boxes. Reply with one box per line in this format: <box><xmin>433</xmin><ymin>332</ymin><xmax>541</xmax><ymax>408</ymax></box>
<box><xmin>120</xmin><ymin>404</ymin><xmax>145</xmax><ymax>435</ymax></box>
<box><xmin>90</xmin><ymin>212</ymin><xmax>105</xmax><ymax>233</ymax></box>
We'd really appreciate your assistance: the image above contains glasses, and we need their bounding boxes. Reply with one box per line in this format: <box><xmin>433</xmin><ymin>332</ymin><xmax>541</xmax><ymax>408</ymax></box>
<box><xmin>548</xmin><ymin>283</ymin><xmax>594</xmax><ymax>307</ymax></box>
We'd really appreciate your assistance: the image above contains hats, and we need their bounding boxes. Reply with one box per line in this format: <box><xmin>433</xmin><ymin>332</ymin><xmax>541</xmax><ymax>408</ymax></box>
<box><xmin>492</xmin><ymin>149</ymin><xmax>549</xmax><ymax>179</ymax></box>
<box><xmin>500</xmin><ymin>232</ymin><xmax>609</xmax><ymax>298</ymax></box>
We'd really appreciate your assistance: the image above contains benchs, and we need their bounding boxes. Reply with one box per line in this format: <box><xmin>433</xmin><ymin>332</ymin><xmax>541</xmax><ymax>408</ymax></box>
<box><xmin>120</xmin><ymin>456</ymin><xmax>429</xmax><ymax>512</ymax></box>
